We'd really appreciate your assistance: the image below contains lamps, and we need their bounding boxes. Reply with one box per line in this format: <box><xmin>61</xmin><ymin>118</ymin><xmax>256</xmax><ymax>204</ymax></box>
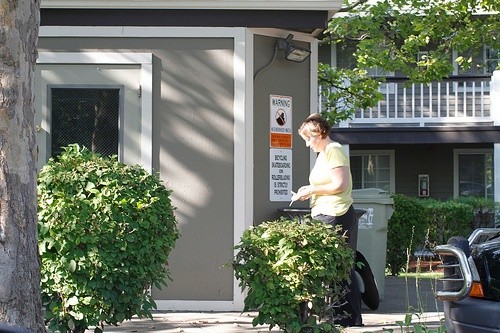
<box><xmin>285</xmin><ymin>45</ymin><xmax>311</xmax><ymax>63</ymax></box>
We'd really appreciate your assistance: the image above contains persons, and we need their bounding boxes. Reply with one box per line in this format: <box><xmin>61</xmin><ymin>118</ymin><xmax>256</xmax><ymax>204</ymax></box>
<box><xmin>290</xmin><ymin>112</ymin><xmax>364</xmax><ymax>327</ymax></box>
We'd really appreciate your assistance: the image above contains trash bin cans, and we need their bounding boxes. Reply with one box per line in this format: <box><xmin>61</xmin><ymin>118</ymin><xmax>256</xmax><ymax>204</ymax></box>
<box><xmin>277</xmin><ymin>208</ymin><xmax>367</xmax><ymax>271</ymax></box>
<box><xmin>350</xmin><ymin>187</ymin><xmax>395</xmax><ymax>304</ymax></box>
